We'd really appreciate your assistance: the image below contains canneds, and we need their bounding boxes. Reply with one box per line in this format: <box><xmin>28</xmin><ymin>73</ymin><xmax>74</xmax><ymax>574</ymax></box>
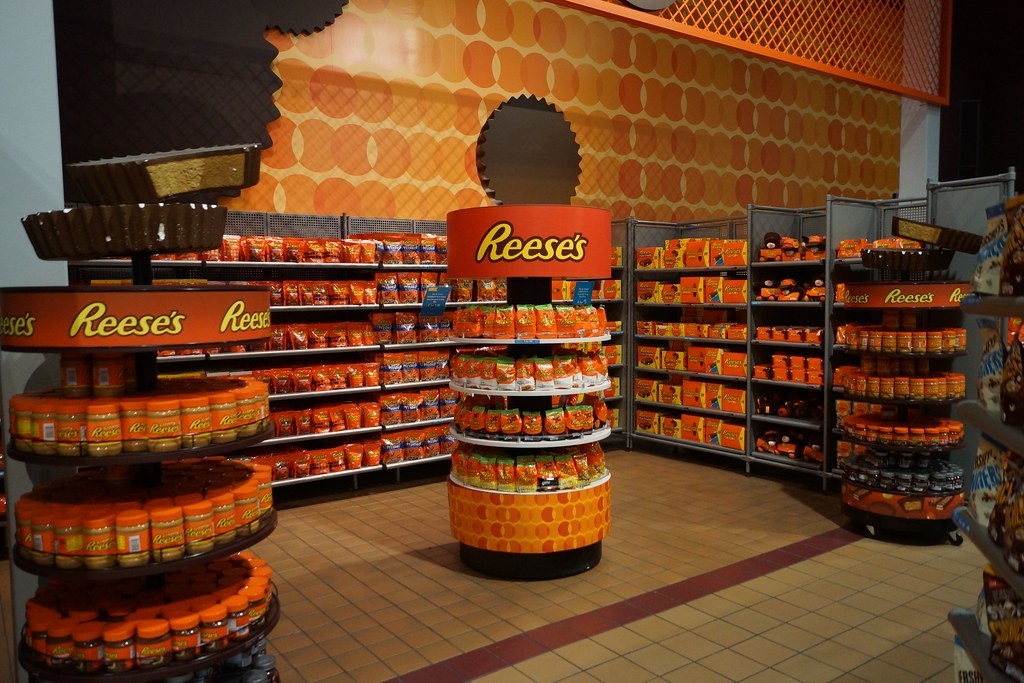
<box><xmin>10</xmin><ymin>378</ymin><xmax>280</xmax><ymax>683</ymax></box>
<box><xmin>845</xmin><ymin>325</ymin><xmax>966</xmax><ymax>494</ymax></box>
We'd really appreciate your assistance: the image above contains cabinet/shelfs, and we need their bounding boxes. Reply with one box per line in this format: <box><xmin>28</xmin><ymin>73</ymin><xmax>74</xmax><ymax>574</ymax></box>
<box><xmin>0</xmin><ymin>260</ymin><xmax>1023</xmax><ymax>683</ymax></box>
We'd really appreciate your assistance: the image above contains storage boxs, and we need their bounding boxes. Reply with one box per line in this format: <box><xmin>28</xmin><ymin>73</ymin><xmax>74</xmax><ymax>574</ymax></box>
<box><xmin>635</xmin><ymin>237</ymin><xmax>748</xmax><ymax>450</ymax></box>
<box><xmin>551</xmin><ymin>247</ymin><xmax>622</xmax><ymax>430</ymax></box>
